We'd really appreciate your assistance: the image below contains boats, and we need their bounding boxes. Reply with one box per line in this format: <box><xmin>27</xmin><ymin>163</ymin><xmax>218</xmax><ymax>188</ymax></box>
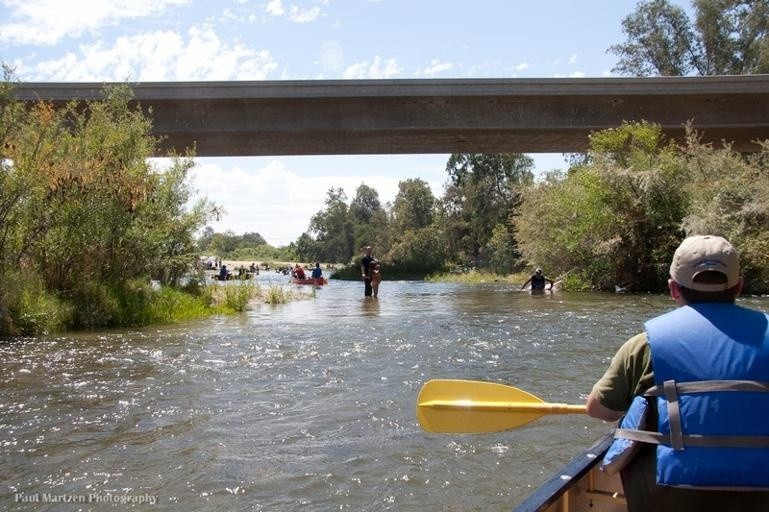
<box><xmin>211</xmin><ymin>272</ymin><xmax>254</xmax><ymax>280</ymax></box>
<box><xmin>510</xmin><ymin>422</ymin><xmax>630</xmax><ymax>512</ymax></box>
<box><xmin>288</xmin><ymin>277</ymin><xmax>327</xmax><ymax>286</ymax></box>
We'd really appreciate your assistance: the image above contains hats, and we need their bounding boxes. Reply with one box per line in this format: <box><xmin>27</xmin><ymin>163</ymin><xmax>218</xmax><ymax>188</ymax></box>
<box><xmin>669</xmin><ymin>234</ymin><xmax>739</xmax><ymax>292</ymax></box>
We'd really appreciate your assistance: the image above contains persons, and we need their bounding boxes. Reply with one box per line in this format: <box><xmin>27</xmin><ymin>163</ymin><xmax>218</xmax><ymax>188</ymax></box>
<box><xmin>587</xmin><ymin>235</ymin><xmax>767</xmax><ymax>510</ymax></box>
<box><xmin>521</xmin><ymin>268</ymin><xmax>554</xmax><ymax>291</ymax></box>
<box><xmin>203</xmin><ymin>246</ymin><xmax>382</xmax><ymax>298</ymax></box>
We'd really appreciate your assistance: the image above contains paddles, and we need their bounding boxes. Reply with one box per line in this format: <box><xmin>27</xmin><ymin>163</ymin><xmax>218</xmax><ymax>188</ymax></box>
<box><xmin>415</xmin><ymin>380</ymin><xmax>586</xmax><ymax>434</ymax></box>
